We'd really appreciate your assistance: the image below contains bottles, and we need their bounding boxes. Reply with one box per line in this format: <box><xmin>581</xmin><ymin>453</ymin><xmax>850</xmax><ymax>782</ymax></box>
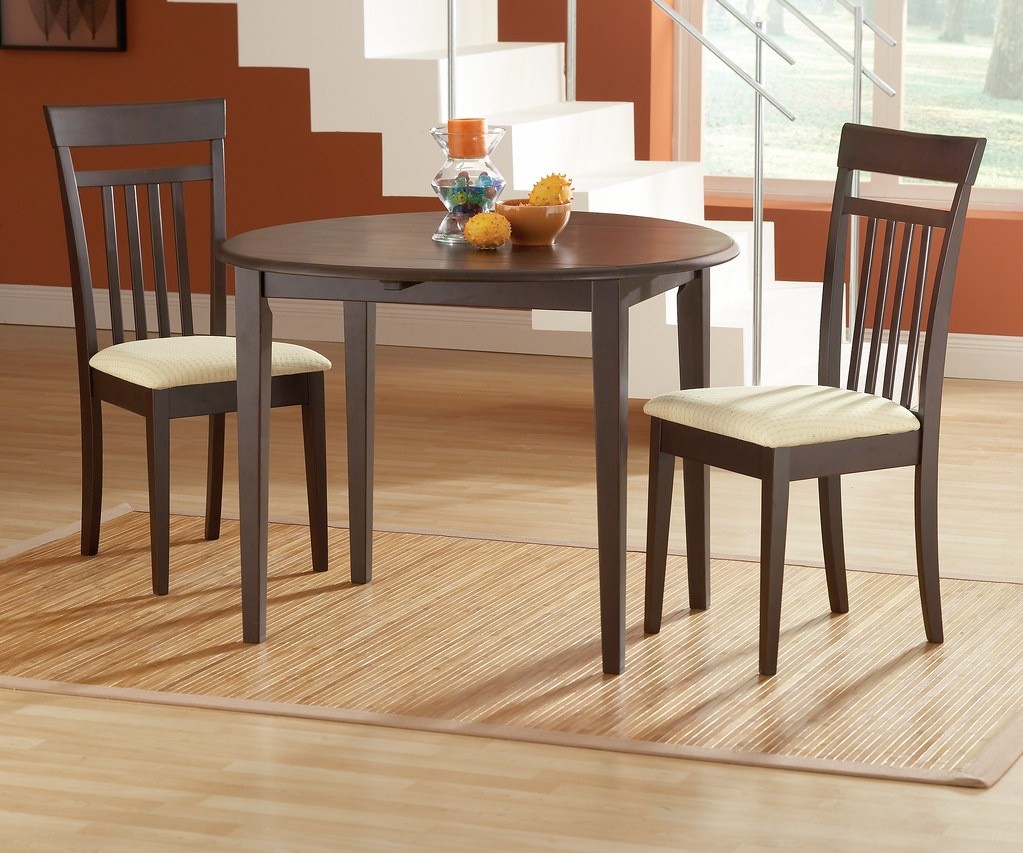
<box><xmin>429</xmin><ymin>119</ymin><xmax>506</xmax><ymax>242</ymax></box>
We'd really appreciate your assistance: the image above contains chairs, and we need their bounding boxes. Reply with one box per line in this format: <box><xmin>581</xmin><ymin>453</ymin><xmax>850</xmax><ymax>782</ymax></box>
<box><xmin>644</xmin><ymin>123</ymin><xmax>989</xmax><ymax>678</ymax></box>
<box><xmin>46</xmin><ymin>98</ymin><xmax>333</xmax><ymax>597</ymax></box>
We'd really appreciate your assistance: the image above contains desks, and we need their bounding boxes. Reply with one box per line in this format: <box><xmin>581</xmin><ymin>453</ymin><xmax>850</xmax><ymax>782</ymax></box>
<box><xmin>217</xmin><ymin>210</ymin><xmax>741</xmax><ymax>672</ymax></box>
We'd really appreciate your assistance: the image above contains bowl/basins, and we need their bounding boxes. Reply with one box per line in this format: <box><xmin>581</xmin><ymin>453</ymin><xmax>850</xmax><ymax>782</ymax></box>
<box><xmin>495</xmin><ymin>198</ymin><xmax>571</xmax><ymax>245</ymax></box>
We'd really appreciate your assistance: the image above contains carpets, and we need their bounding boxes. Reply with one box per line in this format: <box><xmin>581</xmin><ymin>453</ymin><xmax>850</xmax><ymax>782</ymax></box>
<box><xmin>0</xmin><ymin>501</ymin><xmax>1023</xmax><ymax>790</ymax></box>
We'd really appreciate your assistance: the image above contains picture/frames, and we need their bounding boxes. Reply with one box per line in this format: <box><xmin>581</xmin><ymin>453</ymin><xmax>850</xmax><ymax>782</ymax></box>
<box><xmin>0</xmin><ymin>0</ymin><xmax>129</xmax><ymax>52</ymax></box>
